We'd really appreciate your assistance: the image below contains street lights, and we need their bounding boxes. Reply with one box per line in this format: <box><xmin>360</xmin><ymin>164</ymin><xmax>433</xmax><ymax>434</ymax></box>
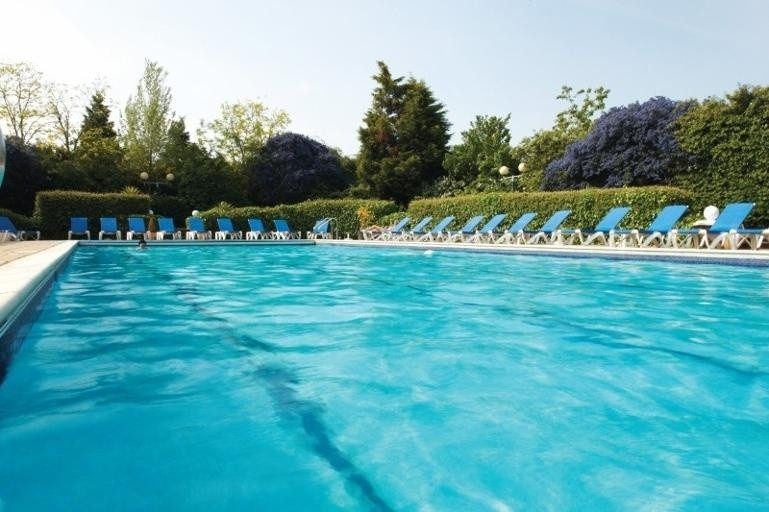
<box><xmin>497</xmin><ymin>162</ymin><xmax>528</xmax><ymax>192</ymax></box>
<box><xmin>136</xmin><ymin>171</ymin><xmax>175</xmax><ymax>192</ymax></box>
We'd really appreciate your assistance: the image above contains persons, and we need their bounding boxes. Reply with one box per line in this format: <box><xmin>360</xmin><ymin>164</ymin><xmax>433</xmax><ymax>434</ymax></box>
<box><xmin>137</xmin><ymin>241</ymin><xmax>147</xmax><ymax>251</ymax></box>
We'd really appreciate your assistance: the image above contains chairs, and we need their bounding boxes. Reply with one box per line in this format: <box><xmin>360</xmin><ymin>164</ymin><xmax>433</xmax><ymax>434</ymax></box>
<box><xmin>0</xmin><ymin>217</ymin><xmax>40</xmax><ymax>241</ymax></box>
